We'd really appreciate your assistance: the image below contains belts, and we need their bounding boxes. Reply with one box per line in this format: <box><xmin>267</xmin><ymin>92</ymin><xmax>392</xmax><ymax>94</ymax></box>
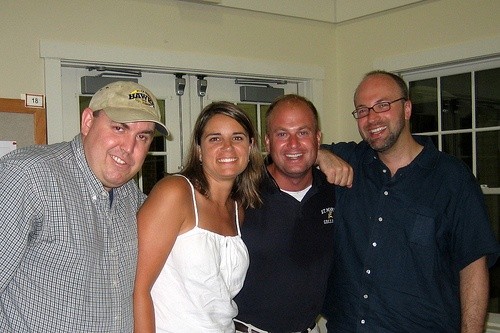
<box><xmin>234</xmin><ymin>320</ymin><xmax>318</xmax><ymax>333</ymax></box>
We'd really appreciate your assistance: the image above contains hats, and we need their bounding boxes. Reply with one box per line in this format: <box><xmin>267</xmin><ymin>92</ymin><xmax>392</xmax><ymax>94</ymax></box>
<box><xmin>87</xmin><ymin>80</ymin><xmax>171</xmax><ymax>138</ymax></box>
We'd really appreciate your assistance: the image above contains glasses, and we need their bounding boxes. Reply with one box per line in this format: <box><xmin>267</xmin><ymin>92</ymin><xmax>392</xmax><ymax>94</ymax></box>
<box><xmin>352</xmin><ymin>98</ymin><xmax>408</xmax><ymax>119</ymax></box>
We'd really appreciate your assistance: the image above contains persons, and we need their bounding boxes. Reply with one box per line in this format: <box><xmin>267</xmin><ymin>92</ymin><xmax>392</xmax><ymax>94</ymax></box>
<box><xmin>0</xmin><ymin>81</ymin><xmax>170</xmax><ymax>333</ymax></box>
<box><xmin>235</xmin><ymin>94</ymin><xmax>336</xmax><ymax>333</ymax></box>
<box><xmin>133</xmin><ymin>101</ymin><xmax>263</xmax><ymax>333</ymax></box>
<box><xmin>264</xmin><ymin>70</ymin><xmax>500</xmax><ymax>333</ymax></box>
